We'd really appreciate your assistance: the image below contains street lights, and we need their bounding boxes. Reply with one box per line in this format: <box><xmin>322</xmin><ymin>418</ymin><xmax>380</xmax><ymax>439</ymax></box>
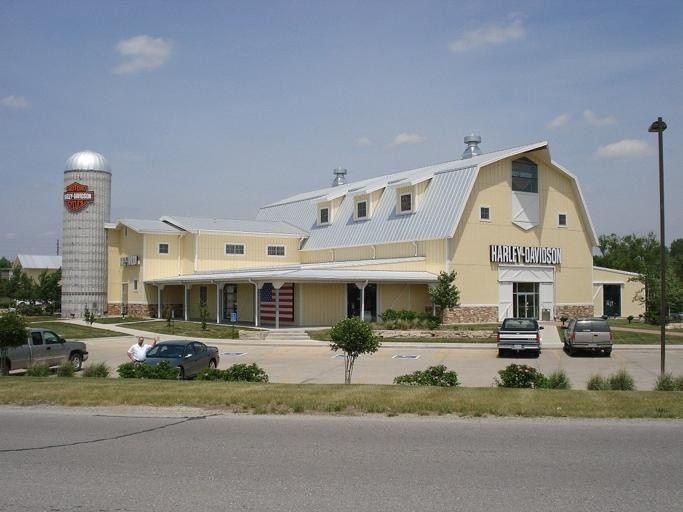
<box><xmin>646</xmin><ymin>116</ymin><xmax>667</xmax><ymax>379</ymax></box>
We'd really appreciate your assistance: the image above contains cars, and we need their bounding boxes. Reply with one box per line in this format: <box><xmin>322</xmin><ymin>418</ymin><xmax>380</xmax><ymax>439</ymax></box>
<box><xmin>138</xmin><ymin>339</ymin><xmax>219</xmax><ymax>382</ymax></box>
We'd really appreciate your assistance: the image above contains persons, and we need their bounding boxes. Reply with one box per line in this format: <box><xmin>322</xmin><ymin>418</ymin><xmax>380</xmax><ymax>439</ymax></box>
<box><xmin>126</xmin><ymin>335</ymin><xmax>160</xmax><ymax>361</ymax></box>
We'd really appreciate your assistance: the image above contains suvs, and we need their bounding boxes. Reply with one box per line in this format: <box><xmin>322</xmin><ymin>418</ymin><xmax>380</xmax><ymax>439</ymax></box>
<box><xmin>560</xmin><ymin>317</ymin><xmax>613</xmax><ymax>357</ymax></box>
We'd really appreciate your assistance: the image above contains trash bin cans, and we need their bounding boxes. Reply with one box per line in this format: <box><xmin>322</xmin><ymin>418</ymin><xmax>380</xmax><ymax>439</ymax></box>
<box><xmin>542</xmin><ymin>308</ymin><xmax>550</xmax><ymax>321</ymax></box>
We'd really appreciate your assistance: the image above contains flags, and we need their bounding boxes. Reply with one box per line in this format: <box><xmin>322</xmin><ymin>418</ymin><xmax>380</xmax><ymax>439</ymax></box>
<box><xmin>259</xmin><ymin>282</ymin><xmax>293</xmax><ymax>322</ymax></box>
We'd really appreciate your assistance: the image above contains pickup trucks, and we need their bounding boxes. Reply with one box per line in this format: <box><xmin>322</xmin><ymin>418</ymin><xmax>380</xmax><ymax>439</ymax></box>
<box><xmin>0</xmin><ymin>328</ymin><xmax>89</xmax><ymax>376</ymax></box>
<box><xmin>497</xmin><ymin>317</ymin><xmax>544</xmax><ymax>357</ymax></box>
